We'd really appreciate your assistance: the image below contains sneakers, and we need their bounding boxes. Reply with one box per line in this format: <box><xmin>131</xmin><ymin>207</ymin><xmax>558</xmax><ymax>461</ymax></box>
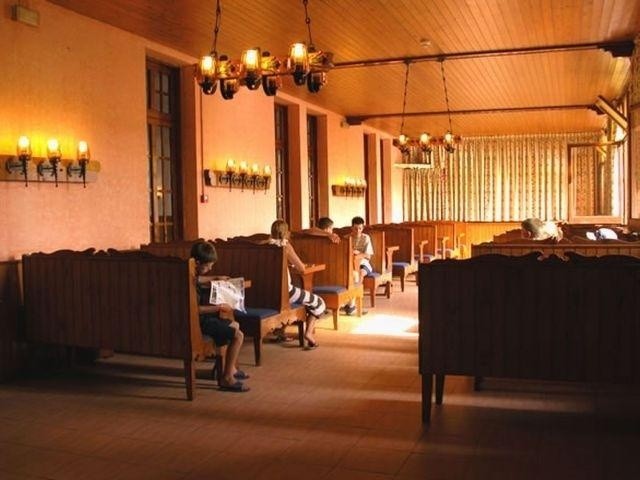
<box><xmin>345</xmin><ymin>305</ymin><xmax>357</xmax><ymax>315</ymax></box>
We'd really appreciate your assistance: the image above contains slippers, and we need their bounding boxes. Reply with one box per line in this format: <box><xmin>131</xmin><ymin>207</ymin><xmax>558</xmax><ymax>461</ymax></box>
<box><xmin>303</xmin><ymin>339</ymin><xmax>321</xmax><ymax>353</ymax></box>
<box><xmin>233</xmin><ymin>366</ymin><xmax>252</xmax><ymax>380</ymax></box>
<box><xmin>275</xmin><ymin>333</ymin><xmax>295</xmax><ymax>343</ymax></box>
<box><xmin>216</xmin><ymin>380</ymin><xmax>251</xmax><ymax>393</ymax></box>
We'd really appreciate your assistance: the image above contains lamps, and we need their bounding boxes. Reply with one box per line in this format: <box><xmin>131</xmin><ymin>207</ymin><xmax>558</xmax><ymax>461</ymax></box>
<box><xmin>340</xmin><ymin>177</ymin><xmax>367</xmax><ymax>197</ymax></box>
<box><xmin>194</xmin><ymin>0</ymin><xmax>336</xmax><ymax>99</ymax></box>
<box><xmin>393</xmin><ymin>56</ymin><xmax>462</xmax><ymax>156</ymax></box>
<box><xmin>3</xmin><ymin>136</ymin><xmax>95</xmax><ymax>188</ymax></box>
<box><xmin>220</xmin><ymin>159</ymin><xmax>271</xmax><ymax>194</ymax></box>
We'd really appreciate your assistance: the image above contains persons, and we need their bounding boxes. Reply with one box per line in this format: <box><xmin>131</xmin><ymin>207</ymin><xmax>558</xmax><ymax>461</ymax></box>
<box><xmin>302</xmin><ymin>217</ymin><xmax>369</xmax><ymax>315</ymax></box>
<box><xmin>189</xmin><ymin>241</ymin><xmax>251</xmax><ymax>392</ymax></box>
<box><xmin>521</xmin><ymin>218</ymin><xmax>618</xmax><ymax>241</ymax></box>
<box><xmin>339</xmin><ymin>216</ymin><xmax>374</xmax><ymax>315</ymax></box>
<box><xmin>253</xmin><ymin>219</ymin><xmax>326</xmax><ymax>350</ymax></box>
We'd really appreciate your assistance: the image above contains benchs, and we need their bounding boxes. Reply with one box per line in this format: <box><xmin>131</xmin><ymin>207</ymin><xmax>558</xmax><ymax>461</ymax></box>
<box><xmin>23</xmin><ymin>247</ymin><xmax>238</xmax><ymax>400</ymax></box>
<box><xmin>142</xmin><ymin>240</ymin><xmax>307</xmax><ymax>365</ymax></box>
<box><xmin>344</xmin><ymin>224</ymin><xmax>418</xmax><ymax>291</ymax></box>
<box><xmin>227</xmin><ymin>230</ymin><xmax>364</xmax><ymax>330</ymax></box>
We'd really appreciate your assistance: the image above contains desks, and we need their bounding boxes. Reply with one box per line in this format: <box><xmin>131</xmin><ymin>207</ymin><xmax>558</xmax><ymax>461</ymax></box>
<box><xmin>302</xmin><ymin>227</ymin><xmax>392</xmax><ymax>307</ymax></box>
<box><xmin>287</xmin><ymin>263</ymin><xmax>326</xmax><ymax>333</ymax></box>
<box><xmin>384</xmin><ymin>245</ymin><xmax>399</xmax><ymax>296</ymax></box>
<box><xmin>351</xmin><ymin>251</ymin><xmax>364</xmax><ymax>283</ymax></box>
<box><xmin>199</xmin><ymin>280</ymin><xmax>252</xmax><ymax>380</ymax></box>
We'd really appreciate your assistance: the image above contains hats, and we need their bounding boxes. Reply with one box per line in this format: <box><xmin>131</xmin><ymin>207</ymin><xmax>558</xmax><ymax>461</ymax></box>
<box><xmin>586</xmin><ymin>228</ymin><xmax>619</xmax><ymax>241</ymax></box>
